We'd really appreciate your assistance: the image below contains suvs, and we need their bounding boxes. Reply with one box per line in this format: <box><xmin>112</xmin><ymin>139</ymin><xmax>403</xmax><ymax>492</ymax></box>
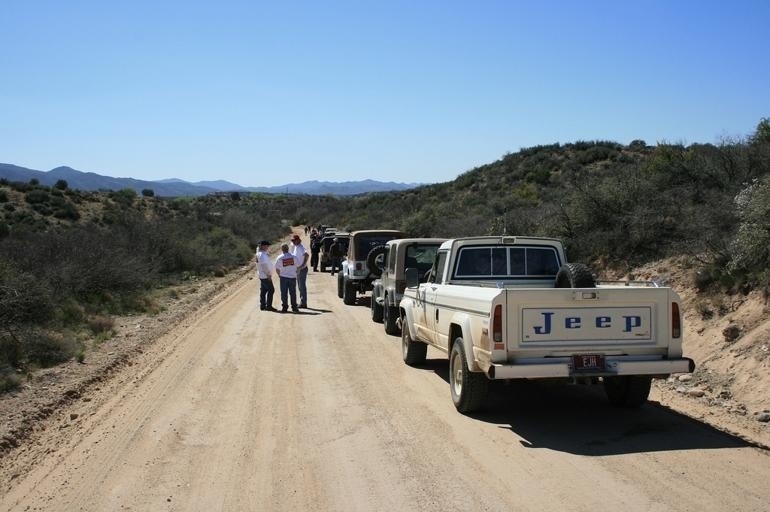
<box><xmin>337</xmin><ymin>229</ymin><xmax>410</xmax><ymax>305</ymax></box>
<box><xmin>318</xmin><ymin>225</ymin><xmax>350</xmax><ymax>273</ymax></box>
<box><xmin>370</xmin><ymin>238</ymin><xmax>456</xmax><ymax>337</ymax></box>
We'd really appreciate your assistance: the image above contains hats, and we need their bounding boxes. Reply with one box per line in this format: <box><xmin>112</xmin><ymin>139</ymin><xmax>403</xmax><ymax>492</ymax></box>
<box><xmin>258</xmin><ymin>240</ymin><xmax>272</xmax><ymax>245</ymax></box>
<box><xmin>291</xmin><ymin>235</ymin><xmax>299</xmax><ymax>240</ymax></box>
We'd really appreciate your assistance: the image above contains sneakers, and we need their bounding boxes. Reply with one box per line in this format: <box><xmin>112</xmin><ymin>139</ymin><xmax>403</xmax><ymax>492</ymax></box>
<box><xmin>261</xmin><ymin>305</ymin><xmax>277</xmax><ymax>311</ymax></box>
<box><xmin>292</xmin><ymin>305</ymin><xmax>299</xmax><ymax>313</ymax></box>
<box><xmin>282</xmin><ymin>304</ymin><xmax>288</xmax><ymax>312</ymax></box>
<box><xmin>298</xmin><ymin>304</ymin><xmax>307</xmax><ymax>308</ymax></box>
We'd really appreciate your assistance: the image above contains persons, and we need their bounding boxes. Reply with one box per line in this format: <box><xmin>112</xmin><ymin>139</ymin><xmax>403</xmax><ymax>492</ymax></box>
<box><xmin>255</xmin><ymin>234</ymin><xmax>309</xmax><ymax>313</ymax></box>
<box><xmin>304</xmin><ymin>223</ymin><xmax>341</xmax><ymax>276</ymax></box>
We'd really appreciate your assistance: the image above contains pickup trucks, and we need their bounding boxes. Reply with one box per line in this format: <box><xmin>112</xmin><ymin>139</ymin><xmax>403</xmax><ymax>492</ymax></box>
<box><xmin>395</xmin><ymin>231</ymin><xmax>696</xmax><ymax>416</ymax></box>
